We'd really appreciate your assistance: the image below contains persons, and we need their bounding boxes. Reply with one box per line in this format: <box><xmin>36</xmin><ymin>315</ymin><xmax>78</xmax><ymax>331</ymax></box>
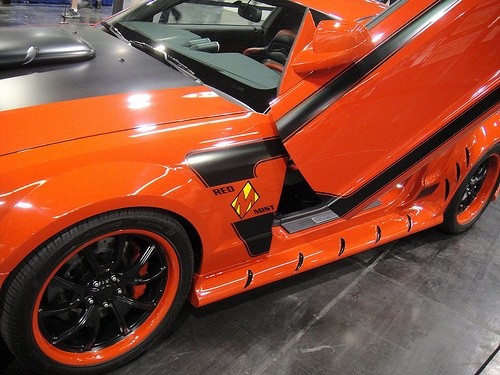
<box><xmin>158</xmin><ymin>6</ymin><xmax>182</xmax><ymax>24</ymax></box>
<box><xmin>61</xmin><ymin>0</ymin><xmax>80</xmax><ymax>18</ymax></box>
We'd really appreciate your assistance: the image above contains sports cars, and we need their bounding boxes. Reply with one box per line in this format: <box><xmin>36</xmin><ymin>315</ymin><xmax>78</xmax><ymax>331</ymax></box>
<box><xmin>0</xmin><ymin>0</ymin><xmax>500</xmax><ymax>375</ymax></box>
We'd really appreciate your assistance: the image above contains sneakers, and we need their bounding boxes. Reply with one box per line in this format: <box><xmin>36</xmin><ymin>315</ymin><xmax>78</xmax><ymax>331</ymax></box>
<box><xmin>61</xmin><ymin>9</ymin><xmax>80</xmax><ymax>19</ymax></box>
